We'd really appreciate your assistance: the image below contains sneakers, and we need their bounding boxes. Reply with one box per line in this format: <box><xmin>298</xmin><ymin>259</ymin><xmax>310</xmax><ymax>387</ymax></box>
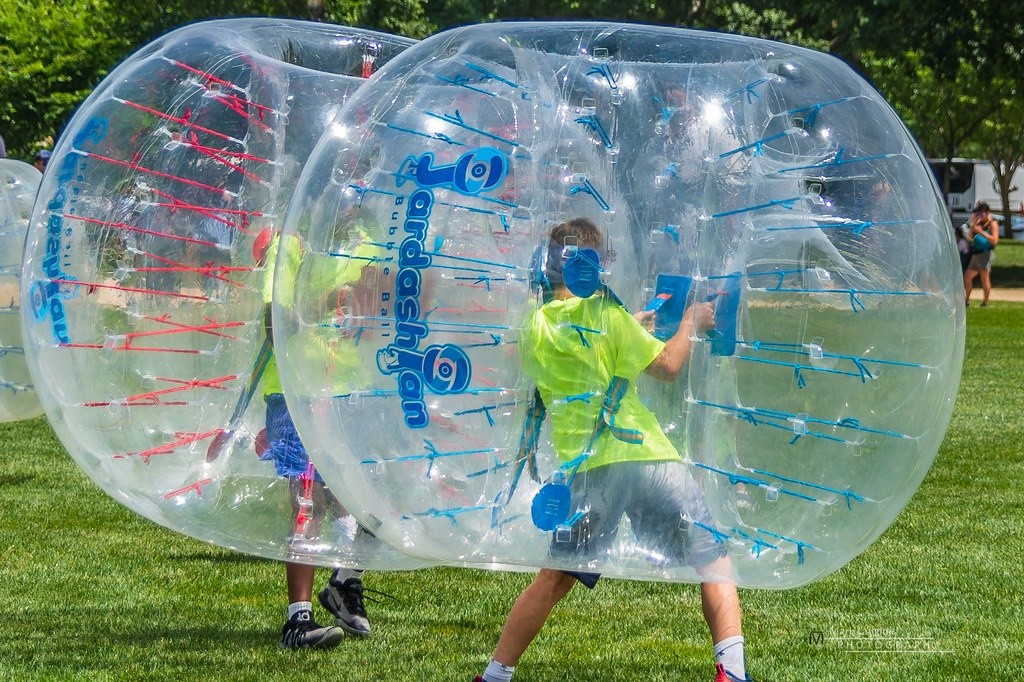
<box><xmin>317</xmin><ymin>572</ymin><xmax>398</xmax><ymax>636</ymax></box>
<box><xmin>280</xmin><ymin>610</ymin><xmax>344</xmax><ymax>652</ymax></box>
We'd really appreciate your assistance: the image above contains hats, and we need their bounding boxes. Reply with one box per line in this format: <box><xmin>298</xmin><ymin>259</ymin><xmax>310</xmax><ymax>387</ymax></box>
<box><xmin>972</xmin><ymin>203</ymin><xmax>989</xmax><ymax>213</ymax></box>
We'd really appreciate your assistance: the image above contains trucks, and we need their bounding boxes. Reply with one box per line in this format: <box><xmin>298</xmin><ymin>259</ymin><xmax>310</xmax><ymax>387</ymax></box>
<box><xmin>925</xmin><ymin>158</ymin><xmax>1024</xmax><ymax>212</ymax></box>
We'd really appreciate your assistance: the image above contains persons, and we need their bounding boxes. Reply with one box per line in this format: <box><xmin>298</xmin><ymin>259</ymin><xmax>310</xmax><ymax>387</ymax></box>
<box><xmin>954</xmin><ymin>203</ymin><xmax>999</xmax><ymax>306</ymax></box>
<box><xmin>264</xmin><ymin>143</ymin><xmax>382</xmax><ymax>650</ymax></box>
<box><xmin>472</xmin><ymin>217</ymin><xmax>754</xmax><ymax>682</ymax></box>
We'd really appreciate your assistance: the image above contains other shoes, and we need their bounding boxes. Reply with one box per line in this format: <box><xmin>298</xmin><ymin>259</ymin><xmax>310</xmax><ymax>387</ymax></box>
<box><xmin>472</xmin><ymin>674</ymin><xmax>487</xmax><ymax>682</ymax></box>
<box><xmin>966</xmin><ymin>301</ymin><xmax>969</xmax><ymax>306</ymax></box>
<box><xmin>713</xmin><ymin>662</ymin><xmax>752</xmax><ymax>682</ymax></box>
<box><xmin>980</xmin><ymin>302</ymin><xmax>988</xmax><ymax>306</ymax></box>
